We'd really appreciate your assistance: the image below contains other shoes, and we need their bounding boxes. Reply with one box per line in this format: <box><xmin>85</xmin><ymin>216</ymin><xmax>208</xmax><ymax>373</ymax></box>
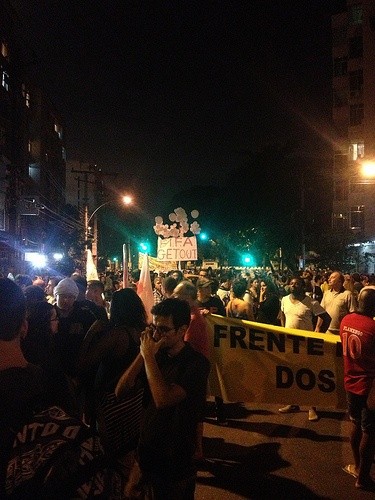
<box><xmin>342</xmin><ymin>464</ymin><xmax>359</xmax><ymax>478</ymax></box>
<box><xmin>309</xmin><ymin>409</ymin><xmax>318</xmax><ymax>420</ymax></box>
<box><xmin>355</xmin><ymin>478</ymin><xmax>374</xmax><ymax>490</ymax></box>
<box><xmin>279</xmin><ymin>404</ymin><xmax>300</xmax><ymax>413</ymax></box>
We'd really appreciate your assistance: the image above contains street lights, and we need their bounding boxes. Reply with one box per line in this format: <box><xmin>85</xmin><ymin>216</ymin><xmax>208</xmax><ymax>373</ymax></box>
<box><xmin>345</xmin><ymin>164</ymin><xmax>375</xmax><ymax>243</ymax></box>
<box><xmin>84</xmin><ymin>196</ymin><xmax>132</xmax><ymax>254</ymax></box>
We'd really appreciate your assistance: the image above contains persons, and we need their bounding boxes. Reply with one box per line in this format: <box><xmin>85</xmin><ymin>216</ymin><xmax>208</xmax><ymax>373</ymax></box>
<box><xmin>0</xmin><ymin>265</ymin><xmax>375</xmax><ymax>500</ymax></box>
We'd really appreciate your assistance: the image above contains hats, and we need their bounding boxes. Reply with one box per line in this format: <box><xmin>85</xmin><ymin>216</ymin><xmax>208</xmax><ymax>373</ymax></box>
<box><xmin>53</xmin><ymin>277</ymin><xmax>79</xmax><ymax>301</ymax></box>
<box><xmin>196</xmin><ymin>277</ymin><xmax>214</xmax><ymax>288</ymax></box>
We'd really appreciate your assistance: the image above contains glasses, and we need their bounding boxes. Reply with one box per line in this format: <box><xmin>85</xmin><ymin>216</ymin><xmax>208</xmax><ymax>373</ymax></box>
<box><xmin>50</xmin><ymin>316</ymin><xmax>59</xmax><ymax>321</ymax></box>
<box><xmin>148</xmin><ymin>322</ymin><xmax>182</xmax><ymax>333</ymax></box>
